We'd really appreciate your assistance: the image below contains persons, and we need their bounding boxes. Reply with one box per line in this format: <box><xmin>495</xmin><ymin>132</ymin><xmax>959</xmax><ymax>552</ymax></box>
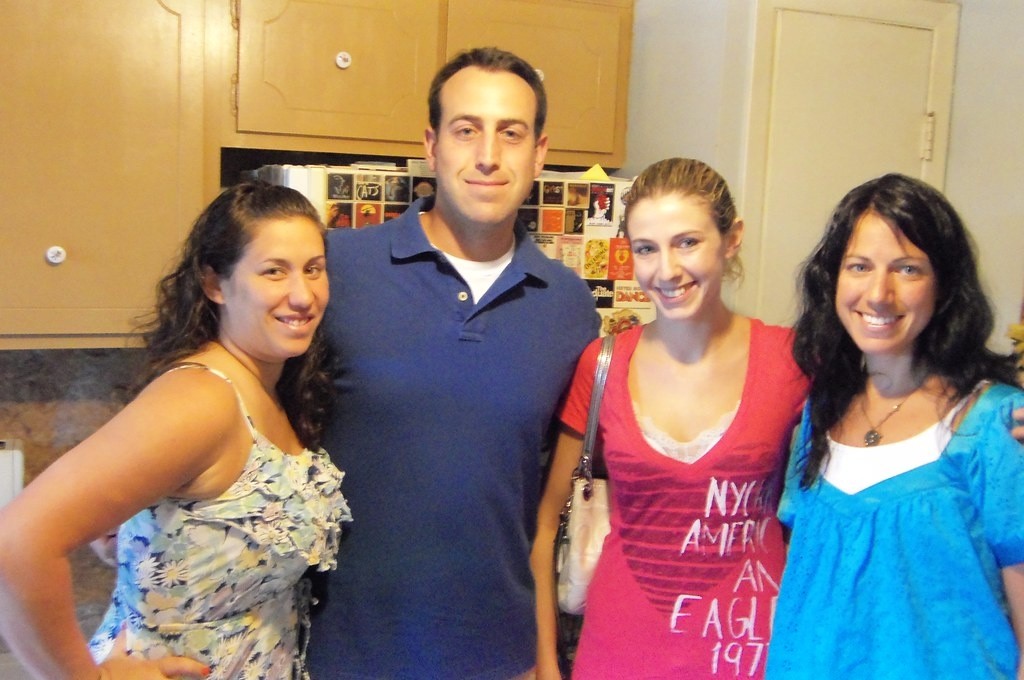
<box><xmin>530</xmin><ymin>159</ymin><xmax>822</xmax><ymax>680</ymax></box>
<box><xmin>277</xmin><ymin>49</ymin><xmax>603</xmax><ymax>680</ymax></box>
<box><xmin>763</xmin><ymin>175</ymin><xmax>1024</xmax><ymax>680</ymax></box>
<box><xmin>0</xmin><ymin>179</ymin><xmax>344</xmax><ymax>680</ymax></box>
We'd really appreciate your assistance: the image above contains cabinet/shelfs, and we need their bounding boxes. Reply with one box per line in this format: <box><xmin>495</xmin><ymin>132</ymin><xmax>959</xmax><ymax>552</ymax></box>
<box><xmin>214</xmin><ymin>1</ymin><xmax>635</xmax><ymax>176</ymax></box>
<box><xmin>1</xmin><ymin>0</ymin><xmax>214</xmax><ymax>349</ymax></box>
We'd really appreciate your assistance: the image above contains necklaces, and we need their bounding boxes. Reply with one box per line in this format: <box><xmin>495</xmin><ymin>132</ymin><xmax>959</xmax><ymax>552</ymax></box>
<box><xmin>860</xmin><ymin>371</ymin><xmax>928</xmax><ymax>447</ymax></box>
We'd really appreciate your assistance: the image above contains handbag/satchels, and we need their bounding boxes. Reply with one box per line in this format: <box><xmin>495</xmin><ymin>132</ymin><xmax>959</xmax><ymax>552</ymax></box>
<box><xmin>556</xmin><ymin>335</ymin><xmax>615</xmax><ymax>614</ymax></box>
